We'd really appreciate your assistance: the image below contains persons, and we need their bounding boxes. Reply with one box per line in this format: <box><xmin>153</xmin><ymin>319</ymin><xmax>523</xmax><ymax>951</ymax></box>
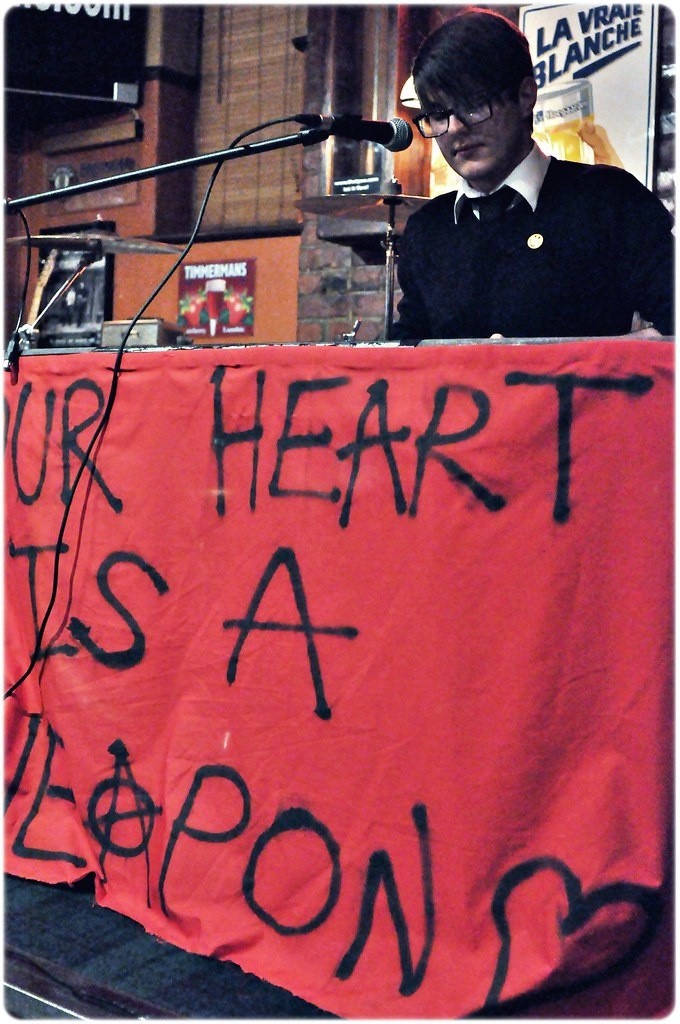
<box><xmin>373</xmin><ymin>10</ymin><xmax>676</xmax><ymax>341</ymax></box>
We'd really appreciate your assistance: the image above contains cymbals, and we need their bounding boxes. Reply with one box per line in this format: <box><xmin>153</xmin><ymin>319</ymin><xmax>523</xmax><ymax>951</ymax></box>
<box><xmin>290</xmin><ymin>193</ymin><xmax>433</xmax><ymax>223</ymax></box>
<box><xmin>5</xmin><ymin>233</ymin><xmax>183</xmax><ymax>254</ymax></box>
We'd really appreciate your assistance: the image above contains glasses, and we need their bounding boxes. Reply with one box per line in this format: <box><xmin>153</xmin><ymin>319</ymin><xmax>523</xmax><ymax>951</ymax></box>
<box><xmin>413</xmin><ymin>82</ymin><xmax>519</xmax><ymax>140</ymax></box>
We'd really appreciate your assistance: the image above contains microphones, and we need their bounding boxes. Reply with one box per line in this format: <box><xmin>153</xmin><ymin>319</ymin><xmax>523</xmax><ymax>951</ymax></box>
<box><xmin>296</xmin><ymin>113</ymin><xmax>413</xmax><ymax>152</ymax></box>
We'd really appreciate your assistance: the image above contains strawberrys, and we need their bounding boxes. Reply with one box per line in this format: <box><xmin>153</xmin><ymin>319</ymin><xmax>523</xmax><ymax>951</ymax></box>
<box><xmin>179</xmin><ymin>289</ymin><xmax>253</xmax><ymax>329</ymax></box>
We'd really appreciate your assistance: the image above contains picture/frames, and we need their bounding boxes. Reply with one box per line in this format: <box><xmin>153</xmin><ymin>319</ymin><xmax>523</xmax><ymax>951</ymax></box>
<box><xmin>36</xmin><ymin>220</ymin><xmax>115</xmax><ymax>347</ymax></box>
